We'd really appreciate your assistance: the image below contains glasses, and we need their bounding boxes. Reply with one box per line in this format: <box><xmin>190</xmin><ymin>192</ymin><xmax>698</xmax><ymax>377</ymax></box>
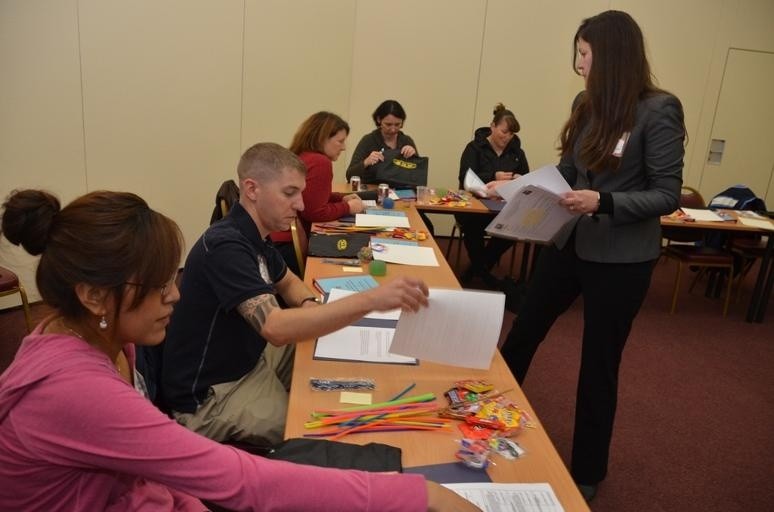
<box><xmin>123</xmin><ymin>268</ymin><xmax>179</xmax><ymax>297</ymax></box>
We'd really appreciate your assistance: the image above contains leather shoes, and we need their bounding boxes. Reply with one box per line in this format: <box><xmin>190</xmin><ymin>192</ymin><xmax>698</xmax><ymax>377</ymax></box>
<box><xmin>576</xmin><ymin>481</ymin><xmax>598</xmax><ymax>502</ymax></box>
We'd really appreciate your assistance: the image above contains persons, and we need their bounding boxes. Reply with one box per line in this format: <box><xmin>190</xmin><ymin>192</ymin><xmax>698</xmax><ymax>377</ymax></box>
<box><xmin>148</xmin><ymin>144</ymin><xmax>429</xmax><ymax>454</ymax></box>
<box><xmin>345</xmin><ymin>100</ymin><xmax>434</xmax><ymax>241</ymax></box>
<box><xmin>0</xmin><ymin>191</ymin><xmax>488</xmax><ymax>512</ymax></box>
<box><xmin>500</xmin><ymin>10</ymin><xmax>688</xmax><ymax>503</ymax></box>
<box><xmin>455</xmin><ymin>104</ymin><xmax>529</xmax><ymax>286</ymax></box>
<box><xmin>269</xmin><ymin>112</ymin><xmax>369</xmax><ymax>276</ymax></box>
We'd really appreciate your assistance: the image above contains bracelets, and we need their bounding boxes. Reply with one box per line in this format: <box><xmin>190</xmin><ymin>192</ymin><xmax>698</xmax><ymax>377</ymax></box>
<box><xmin>597</xmin><ymin>192</ymin><xmax>600</xmax><ymax>206</ymax></box>
<box><xmin>298</xmin><ymin>297</ymin><xmax>320</xmax><ymax>307</ymax></box>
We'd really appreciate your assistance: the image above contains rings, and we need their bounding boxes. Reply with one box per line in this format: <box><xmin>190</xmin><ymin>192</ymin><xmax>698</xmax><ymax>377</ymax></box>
<box><xmin>569</xmin><ymin>205</ymin><xmax>574</xmax><ymax>212</ymax></box>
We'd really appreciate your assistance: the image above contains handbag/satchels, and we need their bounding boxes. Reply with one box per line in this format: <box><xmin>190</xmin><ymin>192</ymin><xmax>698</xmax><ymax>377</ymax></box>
<box><xmin>374</xmin><ymin>147</ymin><xmax>429</xmax><ymax>192</ymax></box>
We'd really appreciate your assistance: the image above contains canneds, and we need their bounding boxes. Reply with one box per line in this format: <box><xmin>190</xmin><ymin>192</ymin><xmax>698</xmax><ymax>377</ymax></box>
<box><xmin>350</xmin><ymin>176</ymin><xmax>360</xmax><ymax>192</ymax></box>
<box><xmin>377</xmin><ymin>184</ymin><xmax>389</xmax><ymax>205</ymax></box>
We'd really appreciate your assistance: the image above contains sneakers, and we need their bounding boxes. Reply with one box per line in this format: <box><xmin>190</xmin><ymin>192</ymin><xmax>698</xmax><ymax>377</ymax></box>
<box><xmin>460</xmin><ymin>263</ymin><xmax>497</xmax><ymax>288</ymax></box>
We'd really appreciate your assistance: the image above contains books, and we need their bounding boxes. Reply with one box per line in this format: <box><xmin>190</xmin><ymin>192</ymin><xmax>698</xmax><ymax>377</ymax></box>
<box><xmin>310</xmin><ymin>273</ymin><xmax>381</xmax><ymax>297</ymax></box>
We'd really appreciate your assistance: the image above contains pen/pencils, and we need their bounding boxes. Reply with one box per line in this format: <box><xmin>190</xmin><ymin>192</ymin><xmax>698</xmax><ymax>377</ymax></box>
<box><xmin>372</xmin><ymin>148</ymin><xmax>385</xmax><ymax>165</ymax></box>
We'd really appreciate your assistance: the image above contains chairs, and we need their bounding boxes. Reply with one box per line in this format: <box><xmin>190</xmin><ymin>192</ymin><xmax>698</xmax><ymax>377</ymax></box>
<box><xmin>0</xmin><ymin>267</ymin><xmax>33</xmax><ymax>350</ymax></box>
<box><xmin>210</xmin><ymin>181</ymin><xmax>239</xmax><ymax>223</ymax></box>
